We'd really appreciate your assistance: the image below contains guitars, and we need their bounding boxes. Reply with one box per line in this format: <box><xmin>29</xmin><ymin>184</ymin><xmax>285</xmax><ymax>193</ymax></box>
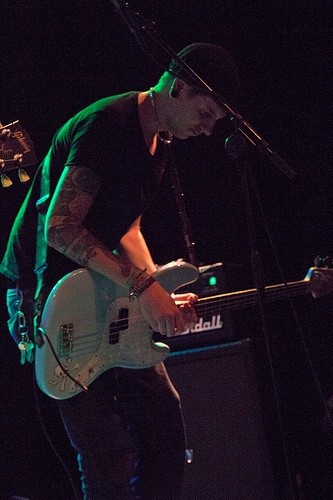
<box><xmin>0</xmin><ymin>119</ymin><xmax>38</xmax><ymax>187</ymax></box>
<box><xmin>35</xmin><ymin>253</ymin><xmax>333</xmax><ymax>400</ymax></box>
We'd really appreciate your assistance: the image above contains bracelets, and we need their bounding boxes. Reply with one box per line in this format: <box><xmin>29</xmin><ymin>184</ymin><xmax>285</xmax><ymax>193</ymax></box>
<box><xmin>128</xmin><ymin>268</ymin><xmax>146</xmax><ymax>291</ymax></box>
<box><xmin>128</xmin><ymin>269</ymin><xmax>157</xmax><ymax>302</ymax></box>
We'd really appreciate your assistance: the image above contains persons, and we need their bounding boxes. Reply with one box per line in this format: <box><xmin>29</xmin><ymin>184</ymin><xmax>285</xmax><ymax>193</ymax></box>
<box><xmin>0</xmin><ymin>41</ymin><xmax>242</xmax><ymax>500</ymax></box>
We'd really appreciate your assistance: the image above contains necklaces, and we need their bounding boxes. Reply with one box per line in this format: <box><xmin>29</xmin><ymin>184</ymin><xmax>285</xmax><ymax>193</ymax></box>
<box><xmin>148</xmin><ymin>87</ymin><xmax>174</xmax><ymax>145</ymax></box>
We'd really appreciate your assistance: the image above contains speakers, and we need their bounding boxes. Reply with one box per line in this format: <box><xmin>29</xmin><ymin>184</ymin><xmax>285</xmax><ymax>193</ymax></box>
<box><xmin>163</xmin><ymin>337</ymin><xmax>278</xmax><ymax>500</ymax></box>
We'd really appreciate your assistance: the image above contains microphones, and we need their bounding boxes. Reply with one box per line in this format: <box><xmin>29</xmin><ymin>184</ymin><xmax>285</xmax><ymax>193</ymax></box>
<box><xmin>114</xmin><ymin>0</ymin><xmax>148</xmax><ymax>55</ymax></box>
